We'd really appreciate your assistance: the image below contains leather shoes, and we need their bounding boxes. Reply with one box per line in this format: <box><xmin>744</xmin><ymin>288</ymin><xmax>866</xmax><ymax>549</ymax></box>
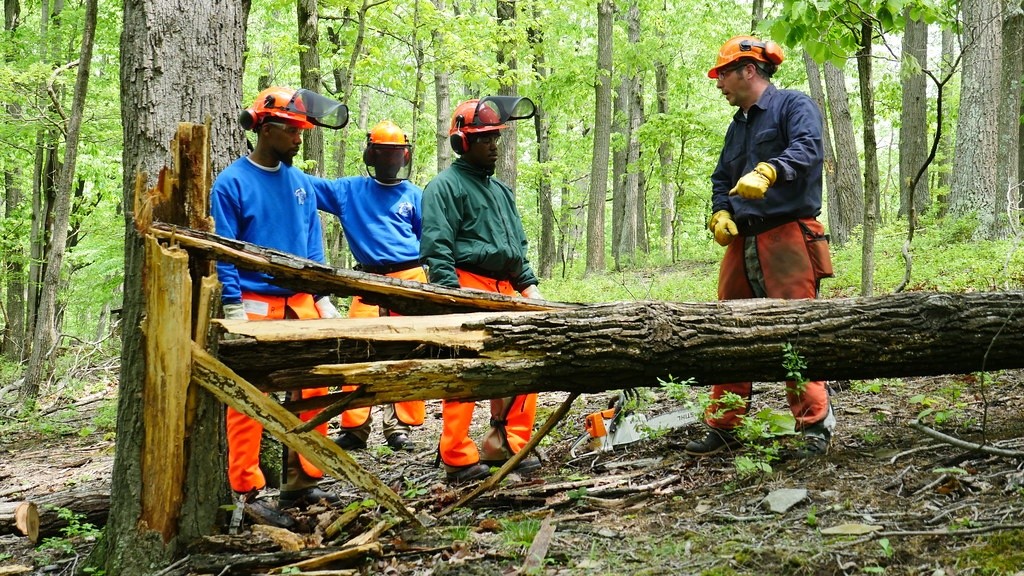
<box><xmin>484</xmin><ymin>455</ymin><xmax>542</xmax><ymax>473</ymax></box>
<box><xmin>278</xmin><ymin>487</ymin><xmax>342</xmax><ymax>512</ymax></box>
<box><xmin>798</xmin><ymin>425</ymin><xmax>831</xmax><ymax>460</ymax></box>
<box><xmin>235</xmin><ymin>491</ymin><xmax>295</xmax><ymax>529</ymax></box>
<box><xmin>380</xmin><ymin>431</ymin><xmax>415</xmax><ymax>451</ymax></box>
<box><xmin>684</xmin><ymin>427</ymin><xmax>744</xmax><ymax>458</ymax></box>
<box><xmin>334</xmin><ymin>421</ymin><xmax>372</xmax><ymax>450</ymax></box>
<box><xmin>446</xmin><ymin>463</ymin><xmax>492</xmax><ymax>484</ymax></box>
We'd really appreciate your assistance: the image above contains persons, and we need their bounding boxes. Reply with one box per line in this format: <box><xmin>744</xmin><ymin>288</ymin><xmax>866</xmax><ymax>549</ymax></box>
<box><xmin>206</xmin><ymin>87</ymin><xmax>349</xmax><ymax>514</ymax></box>
<box><xmin>683</xmin><ymin>35</ymin><xmax>838</xmax><ymax>459</ymax></box>
<box><xmin>300</xmin><ymin>122</ymin><xmax>425</xmax><ymax>450</ymax></box>
<box><xmin>419</xmin><ymin>100</ymin><xmax>548</xmax><ymax>480</ymax></box>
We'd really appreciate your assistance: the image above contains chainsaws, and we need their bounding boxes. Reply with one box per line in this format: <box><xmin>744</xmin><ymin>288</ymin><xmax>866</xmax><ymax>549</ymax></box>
<box><xmin>571</xmin><ymin>388</ymin><xmax>700</xmax><ymax>459</ymax></box>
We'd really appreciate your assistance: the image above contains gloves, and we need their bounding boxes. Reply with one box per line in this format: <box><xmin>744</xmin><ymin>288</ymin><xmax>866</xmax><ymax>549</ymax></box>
<box><xmin>521</xmin><ymin>284</ymin><xmax>545</xmax><ymax>300</ymax></box>
<box><xmin>709</xmin><ymin>210</ymin><xmax>738</xmax><ymax>246</ymax></box>
<box><xmin>314</xmin><ymin>295</ymin><xmax>343</xmax><ymax>319</ymax></box>
<box><xmin>728</xmin><ymin>161</ymin><xmax>778</xmax><ymax>201</ymax></box>
<box><xmin>222</xmin><ymin>303</ymin><xmax>251</xmax><ymax>340</ymax></box>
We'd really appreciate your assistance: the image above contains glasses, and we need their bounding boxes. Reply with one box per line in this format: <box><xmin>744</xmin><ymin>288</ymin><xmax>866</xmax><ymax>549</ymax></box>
<box><xmin>717</xmin><ymin>62</ymin><xmax>749</xmax><ymax>80</ymax></box>
<box><xmin>261</xmin><ymin>121</ymin><xmax>304</xmax><ymax>134</ymax></box>
<box><xmin>468</xmin><ymin>134</ymin><xmax>501</xmax><ymax>145</ymax></box>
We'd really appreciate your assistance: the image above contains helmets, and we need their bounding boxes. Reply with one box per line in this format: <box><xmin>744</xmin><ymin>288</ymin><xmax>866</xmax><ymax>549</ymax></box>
<box><xmin>363</xmin><ymin>121</ymin><xmax>413</xmax><ymax>164</ymax></box>
<box><xmin>707</xmin><ymin>35</ymin><xmax>784</xmax><ymax>79</ymax></box>
<box><xmin>449</xmin><ymin>95</ymin><xmax>508</xmax><ymax>156</ymax></box>
<box><xmin>239</xmin><ymin>86</ymin><xmax>314</xmax><ymax>131</ymax></box>
